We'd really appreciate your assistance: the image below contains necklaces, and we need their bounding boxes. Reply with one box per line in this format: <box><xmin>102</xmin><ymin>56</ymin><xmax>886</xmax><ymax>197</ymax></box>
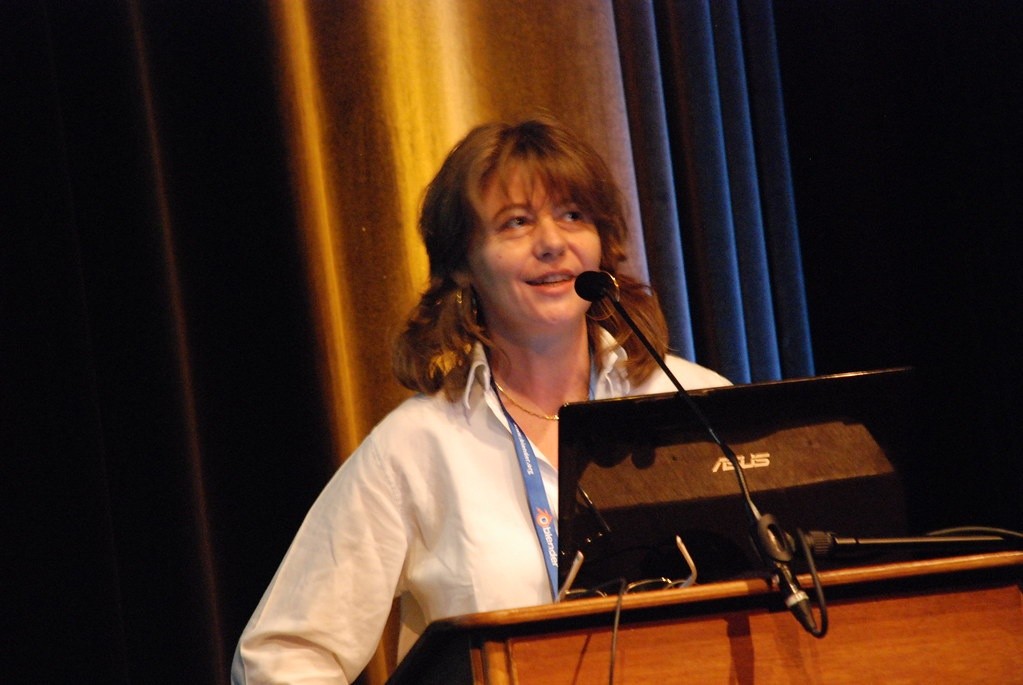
<box><xmin>494</xmin><ymin>380</ymin><xmax>560</xmax><ymax>422</ymax></box>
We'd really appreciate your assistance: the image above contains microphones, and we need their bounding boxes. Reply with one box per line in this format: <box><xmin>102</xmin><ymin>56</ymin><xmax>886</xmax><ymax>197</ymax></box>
<box><xmin>574</xmin><ymin>270</ymin><xmax>816</xmax><ymax>634</ymax></box>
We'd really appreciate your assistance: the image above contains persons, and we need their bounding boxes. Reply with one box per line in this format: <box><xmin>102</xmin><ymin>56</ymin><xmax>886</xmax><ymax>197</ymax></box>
<box><xmin>229</xmin><ymin>114</ymin><xmax>736</xmax><ymax>685</ymax></box>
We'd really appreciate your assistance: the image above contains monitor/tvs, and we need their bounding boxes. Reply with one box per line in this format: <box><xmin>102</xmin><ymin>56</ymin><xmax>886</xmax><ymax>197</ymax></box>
<box><xmin>555</xmin><ymin>365</ymin><xmax>929</xmax><ymax>601</ymax></box>
